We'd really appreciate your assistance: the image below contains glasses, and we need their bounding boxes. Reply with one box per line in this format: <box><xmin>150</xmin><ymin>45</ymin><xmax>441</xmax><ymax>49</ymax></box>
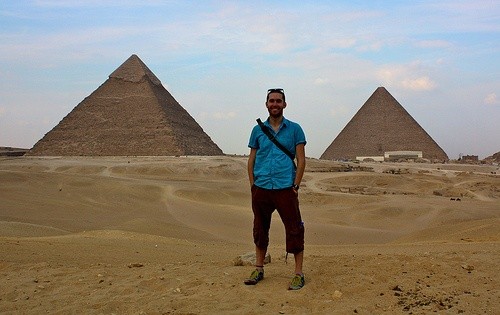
<box><xmin>267</xmin><ymin>89</ymin><xmax>284</xmax><ymax>94</ymax></box>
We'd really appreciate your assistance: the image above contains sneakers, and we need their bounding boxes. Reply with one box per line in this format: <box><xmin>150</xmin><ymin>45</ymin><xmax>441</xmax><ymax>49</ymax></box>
<box><xmin>288</xmin><ymin>272</ymin><xmax>304</xmax><ymax>290</ymax></box>
<box><xmin>244</xmin><ymin>268</ymin><xmax>264</xmax><ymax>285</ymax></box>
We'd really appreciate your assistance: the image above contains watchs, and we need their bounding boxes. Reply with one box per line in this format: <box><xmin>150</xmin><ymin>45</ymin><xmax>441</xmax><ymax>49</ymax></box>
<box><xmin>292</xmin><ymin>183</ymin><xmax>300</xmax><ymax>190</ymax></box>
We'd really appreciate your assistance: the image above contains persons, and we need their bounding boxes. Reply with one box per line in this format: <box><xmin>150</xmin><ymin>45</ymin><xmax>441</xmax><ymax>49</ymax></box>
<box><xmin>244</xmin><ymin>89</ymin><xmax>307</xmax><ymax>291</ymax></box>
